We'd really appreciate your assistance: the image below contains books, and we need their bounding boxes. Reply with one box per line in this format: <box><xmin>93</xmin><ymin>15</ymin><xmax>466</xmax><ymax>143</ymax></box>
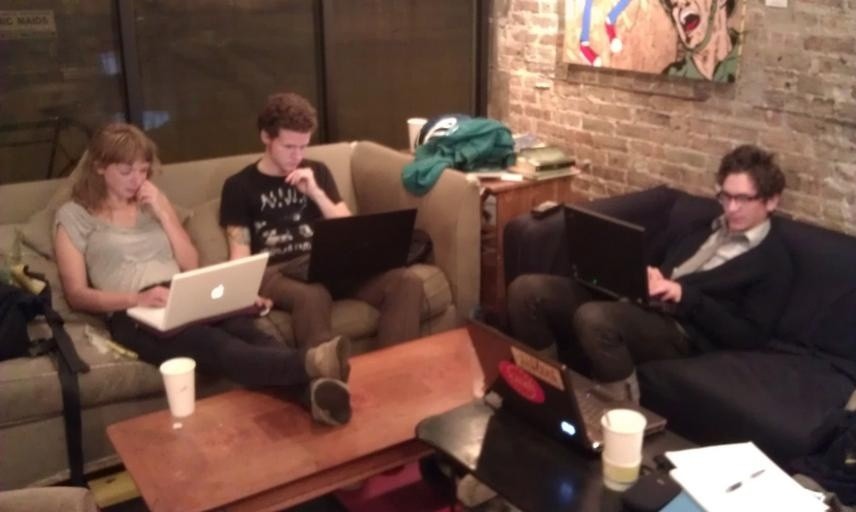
<box><xmin>664</xmin><ymin>440</ymin><xmax>828</xmax><ymax>512</ymax></box>
<box><xmin>492</xmin><ymin>127</ymin><xmax>582</xmax><ymax>183</ymax></box>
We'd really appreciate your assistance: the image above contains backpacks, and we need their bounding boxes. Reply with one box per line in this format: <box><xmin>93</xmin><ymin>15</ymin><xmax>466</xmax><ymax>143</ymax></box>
<box><xmin>0</xmin><ymin>261</ymin><xmax>88</xmax><ymax>486</ymax></box>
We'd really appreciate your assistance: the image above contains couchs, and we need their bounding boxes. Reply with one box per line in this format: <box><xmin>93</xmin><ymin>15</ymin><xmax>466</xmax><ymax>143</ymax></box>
<box><xmin>1</xmin><ymin>137</ymin><xmax>488</xmax><ymax>483</ymax></box>
<box><xmin>501</xmin><ymin>182</ymin><xmax>855</xmax><ymax>470</ymax></box>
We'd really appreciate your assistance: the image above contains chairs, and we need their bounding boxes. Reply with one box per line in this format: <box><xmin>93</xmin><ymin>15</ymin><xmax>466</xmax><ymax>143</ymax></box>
<box><xmin>0</xmin><ymin>484</ymin><xmax>99</xmax><ymax>511</ymax></box>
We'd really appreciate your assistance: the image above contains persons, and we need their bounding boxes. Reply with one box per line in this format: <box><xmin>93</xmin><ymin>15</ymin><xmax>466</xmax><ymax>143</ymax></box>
<box><xmin>215</xmin><ymin>90</ymin><xmax>425</xmax><ymax>355</ymax></box>
<box><xmin>45</xmin><ymin>116</ymin><xmax>359</xmax><ymax>430</ymax></box>
<box><xmin>498</xmin><ymin>141</ymin><xmax>800</xmax><ymax>407</ymax></box>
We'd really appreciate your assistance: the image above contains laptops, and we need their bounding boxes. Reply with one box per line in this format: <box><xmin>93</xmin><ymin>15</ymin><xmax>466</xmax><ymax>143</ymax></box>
<box><xmin>278</xmin><ymin>208</ymin><xmax>418</xmax><ymax>284</ymax></box>
<box><xmin>562</xmin><ymin>203</ymin><xmax>678</xmax><ymax>313</ymax></box>
<box><xmin>464</xmin><ymin>317</ymin><xmax>669</xmax><ymax>456</ymax></box>
<box><xmin>125</xmin><ymin>251</ymin><xmax>271</xmax><ymax>333</ymax></box>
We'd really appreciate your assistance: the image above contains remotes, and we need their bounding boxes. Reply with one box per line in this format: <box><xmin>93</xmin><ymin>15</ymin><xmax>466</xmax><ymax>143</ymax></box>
<box><xmin>530</xmin><ymin>199</ymin><xmax>565</xmax><ymax>218</ymax></box>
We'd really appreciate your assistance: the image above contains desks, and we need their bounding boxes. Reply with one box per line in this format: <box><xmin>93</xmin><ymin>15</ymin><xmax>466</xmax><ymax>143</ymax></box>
<box><xmin>411</xmin><ymin>375</ymin><xmax>838</xmax><ymax>512</ymax></box>
<box><xmin>393</xmin><ymin>151</ymin><xmax>581</xmax><ymax>316</ymax></box>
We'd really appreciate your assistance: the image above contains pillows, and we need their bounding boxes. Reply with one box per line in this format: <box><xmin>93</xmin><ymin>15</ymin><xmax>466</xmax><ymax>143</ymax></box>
<box><xmin>186</xmin><ymin>193</ymin><xmax>235</xmax><ymax>266</ymax></box>
<box><xmin>12</xmin><ymin>147</ymin><xmax>193</xmax><ymax>286</ymax></box>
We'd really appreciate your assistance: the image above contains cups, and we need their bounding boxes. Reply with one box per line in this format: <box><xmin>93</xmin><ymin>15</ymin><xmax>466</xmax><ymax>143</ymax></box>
<box><xmin>600</xmin><ymin>407</ymin><xmax>646</xmax><ymax>491</ymax></box>
<box><xmin>159</xmin><ymin>355</ymin><xmax>197</xmax><ymax>419</ymax></box>
<box><xmin>405</xmin><ymin>117</ymin><xmax>428</xmax><ymax>157</ymax></box>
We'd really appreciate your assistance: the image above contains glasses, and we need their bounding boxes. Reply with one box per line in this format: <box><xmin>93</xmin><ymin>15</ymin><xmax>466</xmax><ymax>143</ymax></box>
<box><xmin>715</xmin><ymin>189</ymin><xmax>763</xmax><ymax>206</ymax></box>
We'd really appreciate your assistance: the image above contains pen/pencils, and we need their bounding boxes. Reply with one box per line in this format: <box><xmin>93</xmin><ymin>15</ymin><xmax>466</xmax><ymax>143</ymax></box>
<box><xmin>727</xmin><ymin>468</ymin><xmax>766</xmax><ymax>492</ymax></box>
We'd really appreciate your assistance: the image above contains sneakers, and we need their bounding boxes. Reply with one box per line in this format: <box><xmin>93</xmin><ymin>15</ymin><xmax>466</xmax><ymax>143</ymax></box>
<box><xmin>304</xmin><ymin>334</ymin><xmax>350</xmax><ymax>384</ymax></box>
<box><xmin>303</xmin><ymin>378</ymin><xmax>353</xmax><ymax>427</ymax></box>
